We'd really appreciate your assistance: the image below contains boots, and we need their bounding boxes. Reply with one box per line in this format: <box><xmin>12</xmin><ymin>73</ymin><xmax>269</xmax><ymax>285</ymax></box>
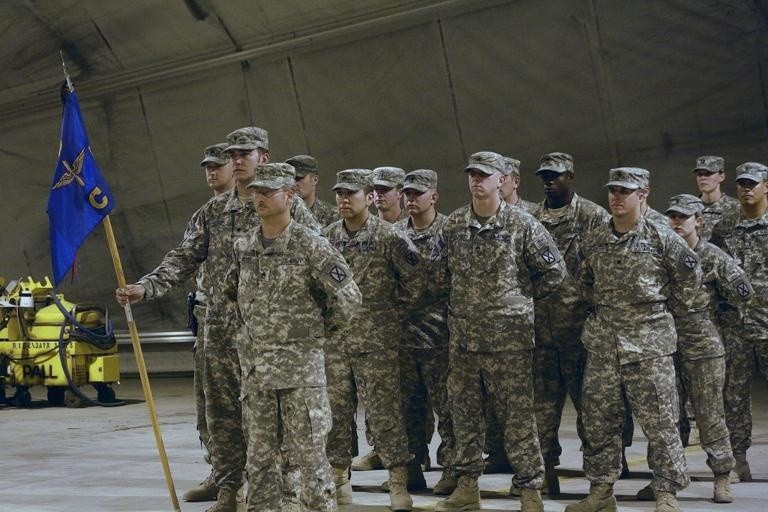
<box><xmin>182</xmin><ymin>468</ymin><xmax>354</xmax><ymax>512</ymax></box>
<box><xmin>564</xmin><ymin>454</ymin><xmax>755</xmax><ymax>511</ymax></box>
<box><xmin>352</xmin><ymin>449</ymin><xmax>561</xmax><ymax>511</ymax></box>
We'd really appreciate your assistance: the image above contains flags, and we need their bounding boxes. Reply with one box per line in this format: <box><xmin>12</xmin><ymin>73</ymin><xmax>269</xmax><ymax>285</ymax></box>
<box><xmin>47</xmin><ymin>84</ymin><xmax>115</xmax><ymax>287</ymax></box>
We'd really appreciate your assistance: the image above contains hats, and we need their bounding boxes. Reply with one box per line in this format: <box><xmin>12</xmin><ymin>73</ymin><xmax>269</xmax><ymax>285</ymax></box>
<box><xmin>533</xmin><ymin>151</ymin><xmax>577</xmax><ymax>176</ymax></box>
<box><xmin>735</xmin><ymin>161</ymin><xmax>768</xmax><ymax>182</ymax></box>
<box><xmin>602</xmin><ymin>166</ymin><xmax>652</xmax><ymax>190</ymax></box>
<box><xmin>690</xmin><ymin>155</ymin><xmax>725</xmax><ymax>173</ymax></box>
<box><xmin>201</xmin><ymin>126</ymin><xmax>320</xmax><ymax>189</ymax></box>
<box><xmin>662</xmin><ymin>194</ymin><xmax>704</xmax><ymax>217</ymax></box>
<box><xmin>331</xmin><ymin>151</ymin><xmax>522</xmax><ymax>194</ymax></box>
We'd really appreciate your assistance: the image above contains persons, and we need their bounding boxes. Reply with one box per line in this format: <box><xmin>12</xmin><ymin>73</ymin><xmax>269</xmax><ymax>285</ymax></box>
<box><xmin>712</xmin><ymin>160</ymin><xmax>766</xmax><ymax>482</ymax></box>
<box><xmin>637</xmin><ymin>193</ymin><xmax>754</xmax><ymax>502</ymax></box>
<box><xmin>237</xmin><ymin>159</ymin><xmax>365</xmax><ymax>511</ymax></box>
<box><xmin>284</xmin><ymin>152</ymin><xmax>339</xmax><ymax>227</ymax></box>
<box><xmin>693</xmin><ymin>154</ymin><xmax>742</xmax><ymax>242</ymax></box>
<box><xmin>566</xmin><ymin>165</ymin><xmax>703</xmax><ymax>511</ymax></box>
<box><xmin>119</xmin><ymin>124</ymin><xmax>325</xmax><ymax>511</ymax></box>
<box><xmin>618</xmin><ymin>170</ymin><xmax>672</xmax><ymax>476</ymax></box>
<box><xmin>182</xmin><ymin>143</ymin><xmax>235</xmax><ymax>502</ymax></box>
<box><xmin>508</xmin><ymin>153</ymin><xmax>612</xmax><ymax>498</ymax></box>
<box><xmin>324</xmin><ymin>169</ymin><xmax>428</xmax><ymax>511</ymax></box>
<box><xmin>353</xmin><ymin>164</ymin><xmax>431</xmax><ymax>471</ymax></box>
<box><xmin>435</xmin><ymin>151</ymin><xmax>566</xmax><ymax>511</ymax></box>
<box><xmin>482</xmin><ymin>156</ymin><xmax>541</xmax><ymax>476</ymax></box>
<box><xmin>379</xmin><ymin>169</ymin><xmax>456</xmax><ymax>494</ymax></box>
